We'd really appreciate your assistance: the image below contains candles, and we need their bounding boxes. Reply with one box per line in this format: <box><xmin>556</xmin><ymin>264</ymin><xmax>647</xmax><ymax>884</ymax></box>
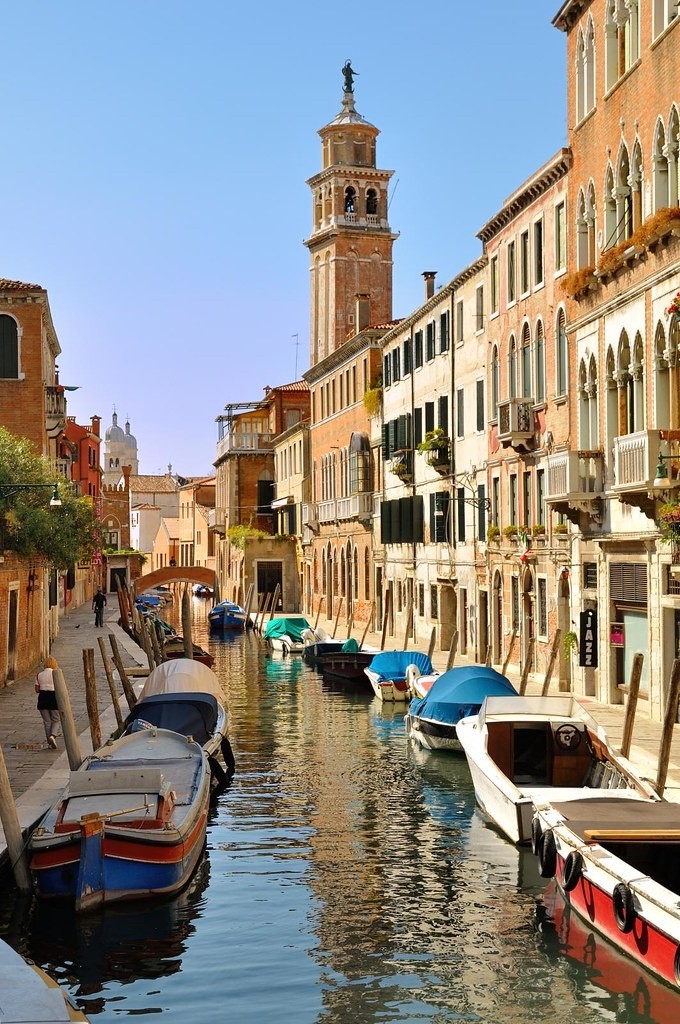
<box><xmin>487</xmin><ymin>526</ymin><xmax>500</xmax><ymax>539</ymax></box>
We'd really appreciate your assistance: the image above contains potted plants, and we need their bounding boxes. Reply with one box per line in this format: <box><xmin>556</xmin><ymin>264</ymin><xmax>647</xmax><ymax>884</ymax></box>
<box><xmin>503</xmin><ymin>525</ymin><xmax>517</xmax><ymax>538</ymax></box>
<box><xmin>532</xmin><ymin>524</ymin><xmax>545</xmax><ymax>536</ymax></box>
<box><xmin>554</xmin><ymin>524</ymin><xmax>567</xmax><ymax>534</ymax></box>
<box><xmin>519</xmin><ymin>524</ymin><xmax>531</xmax><ymax>535</ymax></box>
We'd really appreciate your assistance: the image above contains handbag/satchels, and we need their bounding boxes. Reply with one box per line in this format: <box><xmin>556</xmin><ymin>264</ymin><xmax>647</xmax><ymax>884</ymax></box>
<box><xmin>94</xmin><ymin>606</ymin><xmax>100</xmax><ymax>613</ymax></box>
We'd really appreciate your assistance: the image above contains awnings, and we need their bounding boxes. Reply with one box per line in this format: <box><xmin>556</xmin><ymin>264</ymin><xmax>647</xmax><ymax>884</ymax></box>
<box><xmin>214</xmin><ymin>414</ymin><xmax>233</xmax><ymax>442</ymax></box>
<box><xmin>224</xmin><ymin>401</ymin><xmax>267</xmax><ymax>430</ymax></box>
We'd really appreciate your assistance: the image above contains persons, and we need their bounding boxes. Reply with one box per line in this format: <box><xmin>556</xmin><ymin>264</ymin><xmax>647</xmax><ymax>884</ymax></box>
<box><xmin>92</xmin><ymin>589</ymin><xmax>107</xmax><ymax>627</ymax></box>
<box><xmin>342</xmin><ymin>59</ymin><xmax>360</xmax><ymax>93</ymax></box>
<box><xmin>169</xmin><ymin>555</ymin><xmax>177</xmax><ymax>567</ymax></box>
<box><xmin>34</xmin><ymin>656</ymin><xmax>60</xmax><ymax>749</ymax></box>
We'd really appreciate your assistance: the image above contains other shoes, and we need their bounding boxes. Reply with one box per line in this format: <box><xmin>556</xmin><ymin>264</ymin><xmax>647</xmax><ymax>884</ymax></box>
<box><xmin>49</xmin><ymin>736</ymin><xmax>57</xmax><ymax>749</ymax></box>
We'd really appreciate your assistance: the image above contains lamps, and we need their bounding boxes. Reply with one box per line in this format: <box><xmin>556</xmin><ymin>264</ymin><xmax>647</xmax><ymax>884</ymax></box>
<box><xmin>0</xmin><ymin>483</ymin><xmax>62</xmax><ymax>505</ymax></box>
<box><xmin>653</xmin><ymin>451</ymin><xmax>680</xmax><ymax>487</ymax></box>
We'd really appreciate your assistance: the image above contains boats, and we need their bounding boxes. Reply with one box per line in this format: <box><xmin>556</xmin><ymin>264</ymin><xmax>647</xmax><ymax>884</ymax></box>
<box><xmin>262</xmin><ymin>617</ymin><xmax>332</xmax><ymax>653</ymax></box>
<box><xmin>118</xmin><ymin>586</ymin><xmax>215</xmax><ymax>669</ymax></box>
<box><xmin>363</xmin><ymin>651</ymin><xmax>521</xmax><ymax>752</ymax></box>
<box><xmin>301</xmin><ymin>638</ymin><xmax>386</xmax><ymax>679</ymax></box>
<box><xmin>191</xmin><ymin>583</ymin><xmax>215</xmax><ymax>598</ymax></box>
<box><xmin>209</xmin><ymin>629</ymin><xmax>243</xmax><ymax>644</ymax></box>
<box><xmin>29</xmin><ymin>659</ymin><xmax>230</xmax><ymax>915</ymax></box>
<box><xmin>455</xmin><ymin>696</ymin><xmax>662</xmax><ymax>847</ymax></box>
<box><xmin>531</xmin><ymin>797</ymin><xmax>680</xmax><ymax>1024</ymax></box>
<box><xmin>208</xmin><ymin>600</ymin><xmax>246</xmax><ymax>629</ymax></box>
